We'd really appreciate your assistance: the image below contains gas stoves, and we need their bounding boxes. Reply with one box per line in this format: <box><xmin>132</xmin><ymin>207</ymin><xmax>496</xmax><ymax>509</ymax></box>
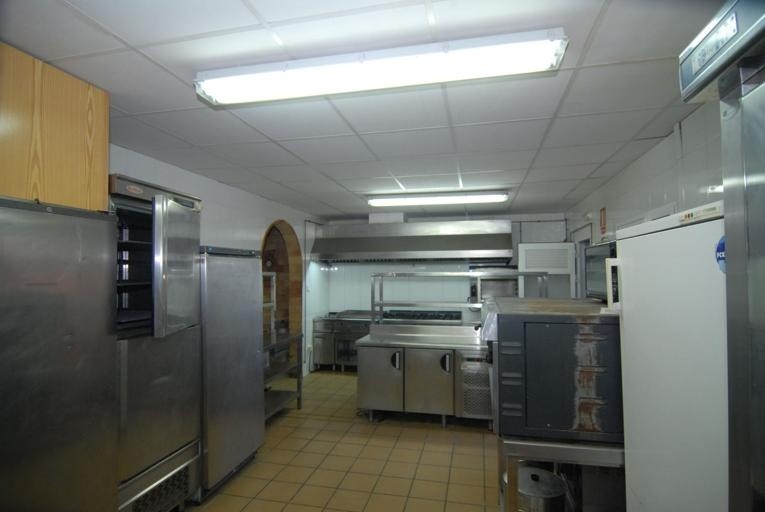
<box><xmin>380</xmin><ymin>309</ymin><xmax>462</xmax><ymax>322</ymax></box>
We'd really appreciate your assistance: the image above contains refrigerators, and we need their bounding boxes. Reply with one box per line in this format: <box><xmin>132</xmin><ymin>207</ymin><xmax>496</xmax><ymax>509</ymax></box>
<box><xmin>615</xmin><ymin>199</ymin><xmax>730</xmax><ymax>512</ymax></box>
<box><xmin>110</xmin><ymin>172</ymin><xmax>201</xmax><ymax>512</ymax></box>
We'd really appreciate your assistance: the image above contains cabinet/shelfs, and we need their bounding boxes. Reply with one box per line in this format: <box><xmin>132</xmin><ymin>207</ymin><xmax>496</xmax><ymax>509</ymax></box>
<box><xmin>0</xmin><ymin>40</ymin><xmax>111</xmax><ymax>216</ymax></box>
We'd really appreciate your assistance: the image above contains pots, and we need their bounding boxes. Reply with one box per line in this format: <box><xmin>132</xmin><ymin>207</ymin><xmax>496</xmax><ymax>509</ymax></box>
<box><xmin>502</xmin><ymin>466</ymin><xmax>566</xmax><ymax>512</ymax></box>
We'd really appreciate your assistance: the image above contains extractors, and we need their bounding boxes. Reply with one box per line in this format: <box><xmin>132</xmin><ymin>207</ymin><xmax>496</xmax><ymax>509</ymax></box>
<box><xmin>310</xmin><ymin>219</ymin><xmax>513</xmax><ymax>263</ymax></box>
